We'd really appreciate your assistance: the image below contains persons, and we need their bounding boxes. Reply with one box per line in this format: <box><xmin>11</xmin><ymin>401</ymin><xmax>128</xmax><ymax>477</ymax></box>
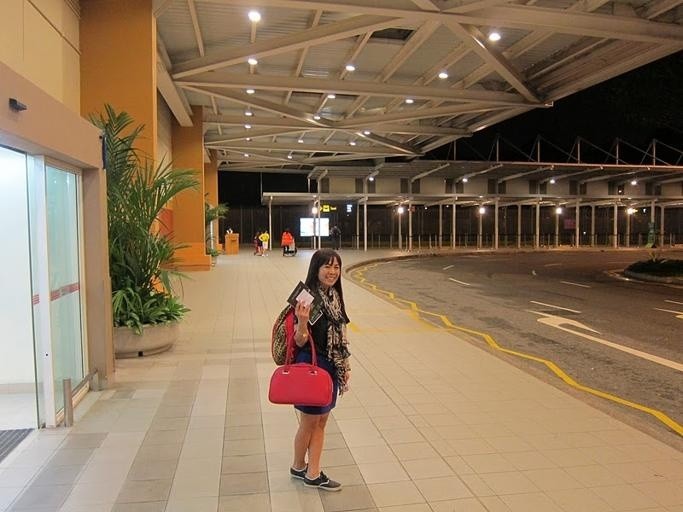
<box><xmin>329</xmin><ymin>223</ymin><xmax>341</xmax><ymax>248</ymax></box>
<box><xmin>280</xmin><ymin>226</ymin><xmax>293</xmax><ymax>257</ymax></box>
<box><xmin>270</xmin><ymin>248</ymin><xmax>351</xmax><ymax>491</ymax></box>
<box><xmin>253</xmin><ymin>232</ymin><xmax>259</xmax><ymax>256</ymax></box>
<box><xmin>259</xmin><ymin>230</ymin><xmax>269</xmax><ymax>257</ymax></box>
<box><xmin>225</xmin><ymin>225</ymin><xmax>235</xmax><ymax>234</ymax></box>
<box><xmin>257</xmin><ymin>231</ymin><xmax>262</xmax><ymax>254</ymax></box>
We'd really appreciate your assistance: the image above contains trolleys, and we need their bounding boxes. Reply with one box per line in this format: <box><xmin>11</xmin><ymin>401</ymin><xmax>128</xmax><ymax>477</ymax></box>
<box><xmin>284</xmin><ymin>237</ymin><xmax>296</xmax><ymax>257</ymax></box>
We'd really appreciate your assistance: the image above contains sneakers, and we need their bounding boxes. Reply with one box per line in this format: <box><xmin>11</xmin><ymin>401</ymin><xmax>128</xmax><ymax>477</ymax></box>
<box><xmin>290</xmin><ymin>463</ymin><xmax>343</xmax><ymax>491</ymax></box>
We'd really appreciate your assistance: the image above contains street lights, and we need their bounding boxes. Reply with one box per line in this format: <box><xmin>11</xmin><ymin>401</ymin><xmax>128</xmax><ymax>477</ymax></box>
<box><xmin>624</xmin><ymin>205</ymin><xmax>632</xmax><ymax>247</ymax></box>
<box><xmin>311</xmin><ymin>199</ymin><xmax>318</xmax><ymax>251</ymax></box>
<box><xmin>478</xmin><ymin>203</ymin><xmax>486</xmax><ymax>248</ymax></box>
<box><xmin>397</xmin><ymin>202</ymin><xmax>404</xmax><ymax>250</ymax></box>
<box><xmin>553</xmin><ymin>205</ymin><xmax>562</xmax><ymax>247</ymax></box>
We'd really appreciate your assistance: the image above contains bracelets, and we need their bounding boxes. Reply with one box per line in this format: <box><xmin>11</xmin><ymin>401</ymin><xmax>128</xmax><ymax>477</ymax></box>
<box><xmin>294</xmin><ymin>328</ymin><xmax>310</xmax><ymax>340</ymax></box>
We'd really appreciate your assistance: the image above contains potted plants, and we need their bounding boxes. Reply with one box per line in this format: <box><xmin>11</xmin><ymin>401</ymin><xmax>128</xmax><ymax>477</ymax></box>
<box><xmin>83</xmin><ymin>102</ymin><xmax>204</xmax><ymax>360</ymax></box>
<box><xmin>204</xmin><ymin>192</ymin><xmax>231</xmax><ymax>267</ymax></box>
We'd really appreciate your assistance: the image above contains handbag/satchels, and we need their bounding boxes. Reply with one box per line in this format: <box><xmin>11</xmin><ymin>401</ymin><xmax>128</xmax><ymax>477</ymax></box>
<box><xmin>268</xmin><ymin>363</ymin><xmax>333</xmax><ymax>407</ymax></box>
<box><xmin>272</xmin><ymin>304</ymin><xmax>300</xmax><ymax>366</ymax></box>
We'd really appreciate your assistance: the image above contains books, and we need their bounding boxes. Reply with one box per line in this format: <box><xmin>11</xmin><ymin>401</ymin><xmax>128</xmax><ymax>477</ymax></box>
<box><xmin>285</xmin><ymin>278</ymin><xmax>325</xmax><ymax>324</ymax></box>
